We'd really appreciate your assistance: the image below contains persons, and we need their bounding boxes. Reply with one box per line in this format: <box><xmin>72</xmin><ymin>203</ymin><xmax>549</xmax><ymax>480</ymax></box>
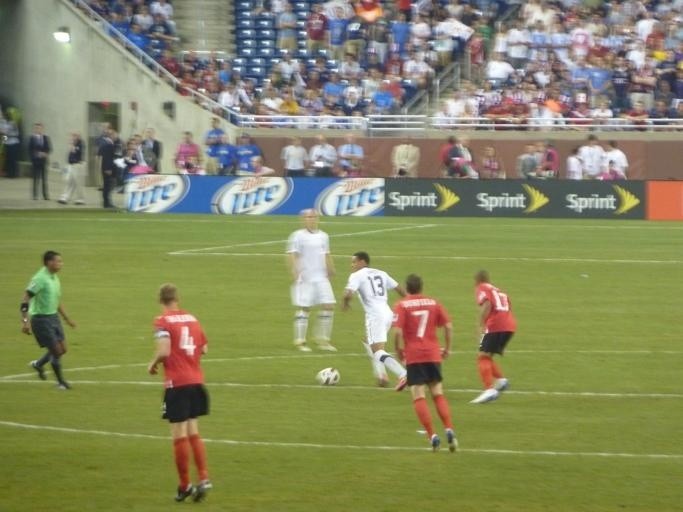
<box><xmin>327</xmin><ymin>6</ymin><xmax>349</xmax><ymax>62</ymax></box>
<box><xmin>479</xmin><ymin>144</ymin><xmax>504</xmax><ymax>179</ymax></box>
<box><xmin>154</xmin><ymin>42</ymin><xmax>180</xmax><ymax>78</ymax></box>
<box><xmin>466</xmin><ymin>1</ymin><xmax>681</xmax><ymax>132</ymax></box>
<box><xmin>235</xmin><ymin>133</ymin><xmax>263</xmax><ymax>175</ymax></box>
<box><xmin>388</xmin><ymin>11</ymin><xmax>413</xmax><ymax>58</ymax></box>
<box><xmin>285</xmin><ymin>206</ymin><xmax>339</xmax><ymax>354</ymax></box>
<box><xmin>179</xmin><ymin>45</ymin><xmax>439</xmax><ymax>116</ymax></box>
<box><xmin>124</xmin><ymin>23</ymin><xmax>150</xmax><ymax>52</ymax></box>
<box><xmin>236</xmin><ymin>155</ymin><xmax>275</xmax><ymax>177</ymax></box>
<box><xmin>87</xmin><ymin>0</ymin><xmax>143</xmax><ymax>37</ymax></box>
<box><xmin>367</xmin><ymin>16</ymin><xmax>393</xmax><ymax>72</ymax></box>
<box><xmin>95</xmin><ymin>120</ymin><xmax>126</xmax><ymax>192</ymax></box>
<box><xmin>174</xmin><ymin>131</ymin><xmax>204</xmax><ymax>175</ymax></box>
<box><xmin>309</xmin><ymin>134</ymin><xmax>337</xmax><ymax>177</ymax></box>
<box><xmin>596</xmin><ymin>138</ymin><xmax>629</xmax><ymax>180</ymax></box>
<box><xmin>147</xmin><ymin>12</ymin><xmax>181</xmax><ymax>51</ymax></box>
<box><xmin>141</xmin><ymin>127</ymin><xmax>162</xmax><ymax>159</ymax></box>
<box><xmin>213</xmin><ymin>132</ymin><xmax>238</xmax><ymax>175</ymax></box>
<box><xmin>389</xmin><ymin>133</ymin><xmax>420</xmax><ymax>177</ymax></box>
<box><xmin>304</xmin><ymin>3</ymin><xmax>329</xmax><ymax>55</ymax></box>
<box><xmin>149</xmin><ymin>0</ymin><xmax>178</xmax><ymax>37</ymax></box>
<box><xmin>466</xmin><ymin>269</ymin><xmax>517</xmax><ymax>405</ymax></box>
<box><xmin>203</xmin><ymin>117</ymin><xmax>227</xmax><ymax>175</ymax></box>
<box><xmin>0</xmin><ymin>95</ymin><xmax>21</xmax><ymax>179</ymax></box>
<box><xmin>130</xmin><ymin>5</ymin><xmax>154</xmax><ymax>34</ymax></box>
<box><xmin>440</xmin><ymin>133</ymin><xmax>481</xmax><ymax>180</ymax></box>
<box><xmin>279</xmin><ymin>135</ymin><xmax>309</xmax><ymax>177</ymax></box>
<box><xmin>274</xmin><ymin>4</ymin><xmax>299</xmax><ymax>61</ymax></box>
<box><xmin>20</xmin><ymin>250</ymin><xmax>77</xmax><ymax>393</ymax></box>
<box><xmin>58</xmin><ymin>128</ymin><xmax>90</xmax><ymax>206</ymax></box>
<box><xmin>330</xmin><ymin>0</ymin><xmax>501</xmax><ymax>22</ymax></box>
<box><xmin>344</xmin><ymin>11</ymin><xmax>367</xmax><ymax>67</ymax></box>
<box><xmin>257</xmin><ymin>0</ymin><xmax>297</xmax><ymax>19</ymax></box>
<box><xmin>29</xmin><ymin>121</ymin><xmax>53</xmax><ymax>200</ymax></box>
<box><xmin>148</xmin><ymin>282</ymin><xmax>214</xmax><ymax>506</ymax></box>
<box><xmin>122</xmin><ymin>134</ymin><xmax>158</xmax><ymax>179</ymax></box>
<box><xmin>580</xmin><ymin>134</ymin><xmax>606</xmax><ymax>179</ymax></box>
<box><xmin>96</xmin><ymin>129</ymin><xmax>118</xmax><ymax>208</ymax></box>
<box><xmin>393</xmin><ymin>275</ymin><xmax>460</xmax><ymax>454</ymax></box>
<box><xmin>564</xmin><ymin>134</ymin><xmax>586</xmax><ymax>180</ymax></box>
<box><xmin>341</xmin><ymin>251</ymin><xmax>411</xmax><ymax>392</ymax></box>
<box><xmin>516</xmin><ymin>140</ymin><xmax>560</xmax><ymax>179</ymax></box>
<box><xmin>410</xmin><ymin>11</ymin><xmax>432</xmax><ymax>53</ymax></box>
<box><xmin>337</xmin><ymin>133</ymin><xmax>364</xmax><ymax>177</ymax></box>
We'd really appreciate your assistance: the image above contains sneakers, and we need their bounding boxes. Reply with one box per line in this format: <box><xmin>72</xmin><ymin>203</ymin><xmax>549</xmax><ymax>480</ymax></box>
<box><xmin>446</xmin><ymin>428</ymin><xmax>459</xmax><ymax>453</ymax></box>
<box><xmin>394</xmin><ymin>377</ymin><xmax>408</xmax><ymax>391</ymax></box>
<box><xmin>297</xmin><ymin>344</ymin><xmax>313</xmax><ymax>353</ymax></box>
<box><xmin>430</xmin><ymin>434</ymin><xmax>441</xmax><ymax>452</ymax></box>
<box><xmin>497</xmin><ymin>378</ymin><xmax>508</xmax><ymax>390</ymax></box>
<box><xmin>378</xmin><ymin>373</ymin><xmax>389</xmax><ymax>387</ymax></box>
<box><xmin>57</xmin><ymin>381</ymin><xmax>71</xmax><ymax>391</ymax></box>
<box><xmin>192</xmin><ymin>480</ymin><xmax>213</xmax><ymax>503</ymax></box>
<box><xmin>29</xmin><ymin>359</ymin><xmax>47</xmax><ymax>381</ymax></box>
<box><xmin>319</xmin><ymin>344</ymin><xmax>337</xmax><ymax>352</ymax></box>
<box><xmin>468</xmin><ymin>389</ymin><xmax>500</xmax><ymax>404</ymax></box>
<box><xmin>174</xmin><ymin>483</ymin><xmax>198</xmax><ymax>503</ymax></box>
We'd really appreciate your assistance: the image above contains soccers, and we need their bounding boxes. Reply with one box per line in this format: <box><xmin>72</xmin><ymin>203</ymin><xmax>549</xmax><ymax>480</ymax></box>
<box><xmin>320</xmin><ymin>367</ymin><xmax>340</xmax><ymax>385</ymax></box>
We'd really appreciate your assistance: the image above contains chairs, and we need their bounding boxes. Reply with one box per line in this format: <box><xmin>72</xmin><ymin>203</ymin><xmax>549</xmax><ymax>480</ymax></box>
<box><xmin>258</xmin><ymin>50</ymin><xmax>275</xmax><ymax>58</ymax></box>
<box><xmin>296</xmin><ymin>50</ymin><xmax>311</xmax><ymax>57</ymax></box>
<box><xmin>259</xmin><ymin>12</ymin><xmax>273</xmax><ymax>20</ymax></box>
<box><xmin>236</xmin><ymin>11</ymin><xmax>257</xmax><ymax>19</ymax></box>
<box><xmin>235</xmin><ymin>19</ymin><xmax>255</xmax><ymax>30</ymax></box>
<box><xmin>277</xmin><ymin>49</ymin><xmax>291</xmax><ymax>58</ymax></box>
<box><xmin>296</xmin><ymin>30</ymin><xmax>308</xmax><ymax>39</ymax></box>
<box><xmin>258</xmin><ymin>41</ymin><xmax>275</xmax><ymax>48</ymax></box>
<box><xmin>325</xmin><ymin>59</ymin><xmax>338</xmax><ymax>67</ymax></box>
<box><xmin>252</xmin><ymin>0</ymin><xmax>270</xmax><ymax>10</ymax></box>
<box><xmin>233</xmin><ymin>58</ymin><xmax>245</xmax><ymax>65</ymax></box>
<box><xmin>292</xmin><ymin>1</ymin><xmax>311</xmax><ymax>11</ymax></box>
<box><xmin>319</xmin><ymin>48</ymin><xmax>326</xmax><ymax>56</ymax></box>
<box><xmin>307</xmin><ymin>59</ymin><xmax>317</xmax><ymax>65</ymax></box>
<box><xmin>256</xmin><ymin>20</ymin><xmax>274</xmax><ymax>29</ymax></box>
<box><xmin>247</xmin><ymin>67</ymin><xmax>265</xmax><ymax>78</ymax></box>
<box><xmin>235</xmin><ymin>0</ymin><xmax>254</xmax><ymax>11</ymax></box>
<box><xmin>238</xmin><ymin>47</ymin><xmax>256</xmax><ymax>57</ymax></box>
<box><xmin>232</xmin><ymin>66</ymin><xmax>246</xmax><ymax>77</ymax></box>
<box><xmin>258</xmin><ymin>30</ymin><xmax>276</xmax><ymax>40</ymax></box>
<box><xmin>246</xmin><ymin>58</ymin><xmax>266</xmax><ymax>67</ymax></box>
<box><xmin>296</xmin><ymin>12</ymin><xmax>311</xmax><ymax>20</ymax></box>
<box><xmin>296</xmin><ymin>21</ymin><xmax>308</xmax><ymax>29</ymax></box>
<box><xmin>236</xmin><ymin>29</ymin><xmax>256</xmax><ymax>40</ymax></box>
<box><xmin>235</xmin><ymin>39</ymin><xmax>257</xmax><ymax>49</ymax></box>
<box><xmin>296</xmin><ymin>41</ymin><xmax>309</xmax><ymax>48</ymax></box>
<box><xmin>268</xmin><ymin>58</ymin><xmax>282</xmax><ymax>65</ymax></box>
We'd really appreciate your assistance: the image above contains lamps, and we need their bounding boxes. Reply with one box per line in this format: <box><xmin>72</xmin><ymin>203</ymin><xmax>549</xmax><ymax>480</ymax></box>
<box><xmin>53</xmin><ymin>25</ymin><xmax>70</xmax><ymax>44</ymax></box>
